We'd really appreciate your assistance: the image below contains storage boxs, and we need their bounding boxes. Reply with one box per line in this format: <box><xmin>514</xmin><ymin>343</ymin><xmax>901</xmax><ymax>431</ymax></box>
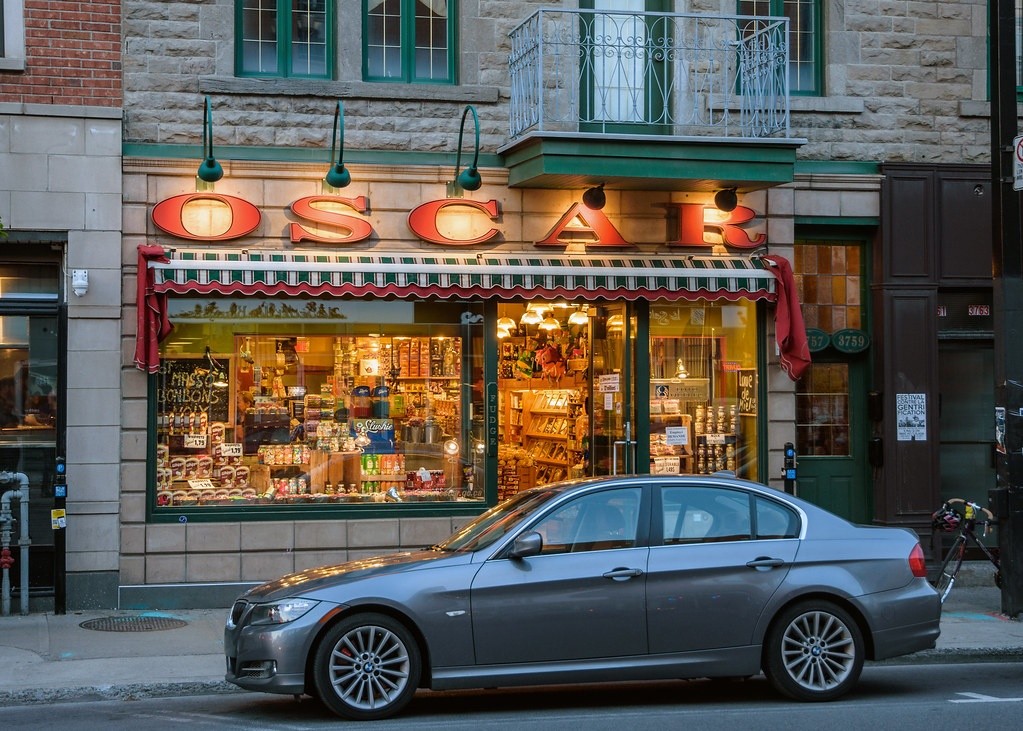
<box><xmin>157</xmin><ymin>337</ymin><xmax>684</xmax><ymax>506</ymax></box>
<box><xmin>693</xmin><ymin>432</ymin><xmax>742</xmax><ymax>479</ymax></box>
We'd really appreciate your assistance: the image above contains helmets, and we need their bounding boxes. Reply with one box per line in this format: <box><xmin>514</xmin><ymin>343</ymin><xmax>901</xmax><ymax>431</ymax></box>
<box><xmin>931</xmin><ymin>507</ymin><xmax>961</xmax><ymax>532</ymax></box>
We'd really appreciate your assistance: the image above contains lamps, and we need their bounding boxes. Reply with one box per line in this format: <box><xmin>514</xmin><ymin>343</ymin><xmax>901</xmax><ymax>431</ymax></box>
<box><xmin>714</xmin><ymin>186</ymin><xmax>738</xmax><ymax>213</ymax></box>
<box><xmin>444</xmin><ymin>104</ymin><xmax>483</xmax><ymax>199</ymax></box>
<box><xmin>445</xmin><ymin>438</ymin><xmax>459</xmax><ymax>492</ymax></box>
<box><xmin>321</xmin><ymin>100</ymin><xmax>351</xmax><ymax>196</ymax></box>
<box><xmin>497</xmin><ymin>303</ymin><xmax>517</xmax><ymax>330</ymax></box>
<box><xmin>497</xmin><ymin>329</ymin><xmax>511</xmax><ymax>338</ymax></box>
<box><xmin>196</xmin><ymin>95</ymin><xmax>224</xmax><ymax>192</ymax></box>
<box><xmin>582</xmin><ymin>182</ymin><xmax>606</xmax><ymax>210</ymax></box>
<box><xmin>520</xmin><ymin>312</ymin><xmax>543</xmax><ymax>324</ymax></box>
<box><xmin>526</xmin><ymin>303</ymin><xmax>554</xmax><ymax>315</ymax></box>
<box><xmin>538</xmin><ymin>313</ymin><xmax>562</xmax><ymax>331</ymax></box>
<box><xmin>606</xmin><ymin>312</ymin><xmax>624</xmax><ymax>325</ymax></box>
<box><xmin>608</xmin><ymin>326</ymin><xmax>622</xmax><ymax>332</ymax></box>
<box><xmin>567</xmin><ymin>306</ymin><xmax>588</xmax><ymax>325</ymax></box>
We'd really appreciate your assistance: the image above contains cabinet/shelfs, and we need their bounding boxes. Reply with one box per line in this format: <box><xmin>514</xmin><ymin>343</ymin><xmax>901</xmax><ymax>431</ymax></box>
<box><xmin>650</xmin><ymin>414</ymin><xmax>693</xmax><ymax>475</ymax></box>
<box><xmin>243</xmin><ymin>423</ymin><xmax>290</xmax><ymax>457</ymax></box>
<box><xmin>387</xmin><ymin>376</ymin><xmax>460</xmax><ymax>407</ymax></box>
<box><xmin>498</xmin><ymin>377</ymin><xmax>583</xmax><ymax>483</ymax></box>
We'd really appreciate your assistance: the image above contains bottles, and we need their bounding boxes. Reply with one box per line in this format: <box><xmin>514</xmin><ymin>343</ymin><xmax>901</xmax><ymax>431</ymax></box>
<box><xmin>362</xmin><ymin>482</ymin><xmax>405</xmax><ymax>494</ymax></box>
<box><xmin>361</xmin><ymin>454</ymin><xmax>405</xmax><ymax>475</ymax></box>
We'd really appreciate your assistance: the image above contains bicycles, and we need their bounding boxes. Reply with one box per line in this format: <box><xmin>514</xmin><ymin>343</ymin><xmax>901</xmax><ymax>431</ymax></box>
<box><xmin>930</xmin><ymin>497</ymin><xmax>1002</xmax><ymax>603</ymax></box>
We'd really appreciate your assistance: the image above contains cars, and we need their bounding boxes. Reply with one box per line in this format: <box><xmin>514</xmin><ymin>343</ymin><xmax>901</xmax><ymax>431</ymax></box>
<box><xmin>223</xmin><ymin>473</ymin><xmax>943</xmax><ymax>721</ymax></box>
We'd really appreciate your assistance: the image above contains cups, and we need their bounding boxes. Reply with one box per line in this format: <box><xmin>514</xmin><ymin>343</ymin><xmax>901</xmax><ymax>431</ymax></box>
<box><xmin>401</xmin><ymin>426</ymin><xmax>442</xmax><ymax>444</ymax></box>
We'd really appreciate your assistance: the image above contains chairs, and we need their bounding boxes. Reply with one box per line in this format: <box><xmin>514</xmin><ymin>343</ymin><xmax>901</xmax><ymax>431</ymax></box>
<box><xmin>585</xmin><ymin>504</ymin><xmax>626</xmax><ymax>550</ymax></box>
